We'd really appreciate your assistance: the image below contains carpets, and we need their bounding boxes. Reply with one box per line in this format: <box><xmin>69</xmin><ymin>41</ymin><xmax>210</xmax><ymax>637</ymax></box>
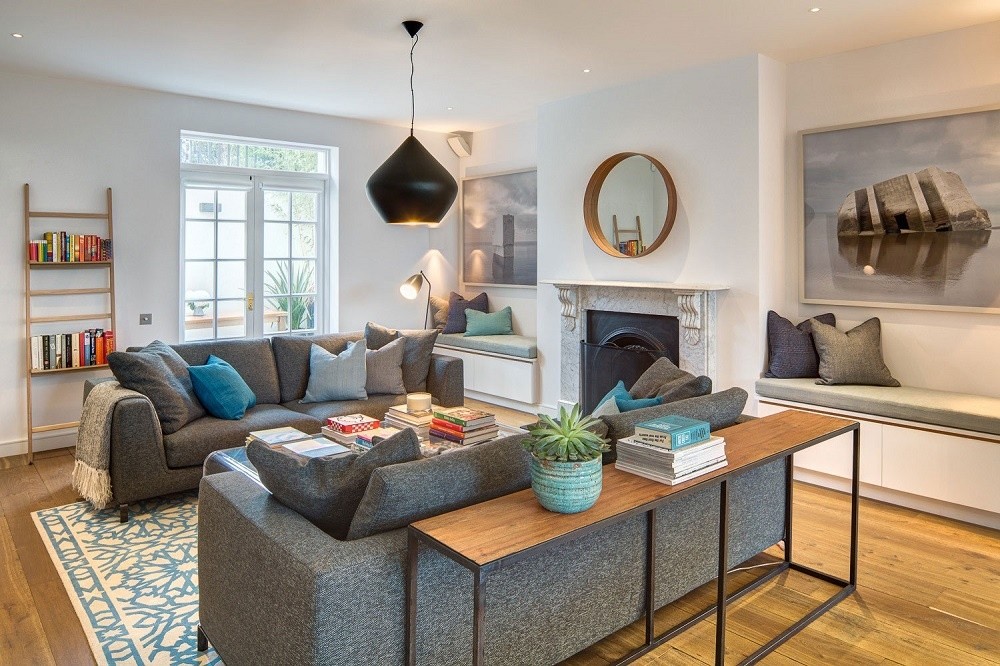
<box><xmin>29</xmin><ymin>502</ymin><xmax>223</xmax><ymax>666</ymax></box>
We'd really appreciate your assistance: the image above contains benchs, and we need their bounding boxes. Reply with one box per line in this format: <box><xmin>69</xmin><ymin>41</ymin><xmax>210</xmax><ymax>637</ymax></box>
<box><xmin>430</xmin><ymin>328</ymin><xmax>538</xmax><ymax>407</ymax></box>
<box><xmin>755</xmin><ymin>311</ymin><xmax>999</xmax><ymax>530</ymax></box>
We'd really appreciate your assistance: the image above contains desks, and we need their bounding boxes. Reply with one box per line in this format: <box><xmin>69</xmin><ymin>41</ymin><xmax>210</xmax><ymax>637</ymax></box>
<box><xmin>185</xmin><ymin>310</ymin><xmax>288</xmax><ymax>332</ymax></box>
<box><xmin>408</xmin><ymin>409</ymin><xmax>861</xmax><ymax>665</ymax></box>
<box><xmin>202</xmin><ymin>423</ymin><xmax>528</xmax><ymax>496</ymax></box>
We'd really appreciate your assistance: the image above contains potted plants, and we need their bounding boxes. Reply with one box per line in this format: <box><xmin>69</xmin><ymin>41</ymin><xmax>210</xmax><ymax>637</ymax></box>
<box><xmin>519</xmin><ymin>402</ymin><xmax>611</xmax><ymax>513</ymax></box>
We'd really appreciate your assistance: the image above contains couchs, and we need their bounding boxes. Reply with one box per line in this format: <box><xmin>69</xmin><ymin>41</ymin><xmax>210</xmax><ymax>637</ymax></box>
<box><xmin>198</xmin><ymin>355</ymin><xmax>784</xmax><ymax>666</ymax></box>
<box><xmin>76</xmin><ymin>323</ymin><xmax>463</xmax><ymax>523</ymax></box>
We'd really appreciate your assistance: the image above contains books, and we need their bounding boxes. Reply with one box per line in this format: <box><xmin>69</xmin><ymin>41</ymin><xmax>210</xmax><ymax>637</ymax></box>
<box><xmin>245</xmin><ymin>427</ymin><xmax>353</xmax><ymax>462</ymax></box>
<box><xmin>30</xmin><ymin>329</ymin><xmax>113</xmax><ymax>371</ymax></box>
<box><xmin>614</xmin><ymin>415</ymin><xmax>729</xmax><ymax>486</ymax></box>
<box><xmin>383</xmin><ymin>403</ymin><xmax>445</xmax><ymax>441</ymax></box>
<box><xmin>29</xmin><ymin>232</ymin><xmax>113</xmax><ymax>262</ymax></box>
<box><xmin>429</xmin><ymin>406</ymin><xmax>499</xmax><ymax>445</ymax></box>
<box><xmin>321</xmin><ymin>426</ymin><xmax>426</xmax><ymax>456</ymax></box>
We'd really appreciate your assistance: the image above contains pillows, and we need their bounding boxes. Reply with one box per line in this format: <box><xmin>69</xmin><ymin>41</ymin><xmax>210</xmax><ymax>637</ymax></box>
<box><xmin>443</xmin><ymin>291</ymin><xmax>488</xmax><ymax>334</ymax></box>
<box><xmin>463</xmin><ymin>305</ymin><xmax>514</xmax><ymax>337</ymax></box>
<box><xmin>105</xmin><ymin>340</ymin><xmax>256</xmax><ymax>433</ymax></box>
<box><xmin>299</xmin><ymin>325</ymin><xmax>438</xmax><ymax>403</ymax></box>
<box><xmin>765</xmin><ymin>311</ymin><xmax>901</xmax><ymax>387</ymax></box>
<box><xmin>592</xmin><ymin>356</ymin><xmax>712</xmax><ymax>419</ymax></box>
<box><xmin>246</xmin><ymin>428</ymin><xmax>421</xmax><ymax>540</ymax></box>
<box><xmin>430</xmin><ymin>297</ymin><xmax>449</xmax><ymax>332</ymax></box>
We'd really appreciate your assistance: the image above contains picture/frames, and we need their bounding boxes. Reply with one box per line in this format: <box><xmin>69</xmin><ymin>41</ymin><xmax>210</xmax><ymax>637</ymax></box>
<box><xmin>798</xmin><ymin>102</ymin><xmax>1000</xmax><ymax>314</ymax></box>
<box><xmin>457</xmin><ymin>167</ymin><xmax>537</xmax><ymax>288</ymax></box>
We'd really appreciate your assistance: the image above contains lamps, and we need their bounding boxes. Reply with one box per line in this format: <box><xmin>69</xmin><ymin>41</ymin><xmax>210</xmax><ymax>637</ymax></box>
<box><xmin>400</xmin><ymin>269</ymin><xmax>431</xmax><ymax>330</ymax></box>
<box><xmin>365</xmin><ymin>21</ymin><xmax>458</xmax><ymax>223</ymax></box>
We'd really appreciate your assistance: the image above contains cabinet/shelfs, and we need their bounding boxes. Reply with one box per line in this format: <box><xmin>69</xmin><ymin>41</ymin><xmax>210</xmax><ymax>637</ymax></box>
<box><xmin>25</xmin><ymin>183</ymin><xmax>116</xmax><ymax>466</ymax></box>
<box><xmin>613</xmin><ymin>215</ymin><xmax>642</xmax><ymax>251</ymax></box>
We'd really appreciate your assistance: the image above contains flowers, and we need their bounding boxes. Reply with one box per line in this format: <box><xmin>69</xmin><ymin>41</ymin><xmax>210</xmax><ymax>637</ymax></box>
<box><xmin>185</xmin><ymin>291</ymin><xmax>211</xmax><ymax>309</ymax></box>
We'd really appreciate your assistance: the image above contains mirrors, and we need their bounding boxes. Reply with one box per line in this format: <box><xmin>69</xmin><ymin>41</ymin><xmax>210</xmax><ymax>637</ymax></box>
<box><xmin>582</xmin><ymin>151</ymin><xmax>676</xmax><ymax>257</ymax></box>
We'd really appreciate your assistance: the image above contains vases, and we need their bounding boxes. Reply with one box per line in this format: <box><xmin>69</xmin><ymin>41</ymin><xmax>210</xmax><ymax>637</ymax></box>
<box><xmin>192</xmin><ymin>307</ymin><xmax>204</xmax><ymax>316</ymax></box>
